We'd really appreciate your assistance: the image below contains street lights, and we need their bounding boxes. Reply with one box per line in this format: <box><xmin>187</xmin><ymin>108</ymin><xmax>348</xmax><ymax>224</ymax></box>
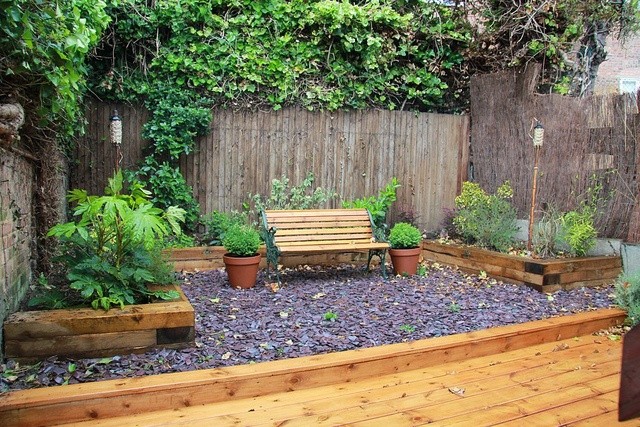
<box><xmin>527</xmin><ymin>121</ymin><xmax>544</xmax><ymax>251</ymax></box>
<box><xmin>109</xmin><ymin>110</ymin><xmax>123</xmax><ymax>249</ymax></box>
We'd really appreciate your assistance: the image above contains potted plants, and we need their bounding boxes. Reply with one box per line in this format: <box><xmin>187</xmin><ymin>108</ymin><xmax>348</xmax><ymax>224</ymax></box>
<box><xmin>389</xmin><ymin>220</ymin><xmax>422</xmax><ymax>277</ymax></box>
<box><xmin>207</xmin><ymin>200</ymin><xmax>260</xmax><ymax>289</ymax></box>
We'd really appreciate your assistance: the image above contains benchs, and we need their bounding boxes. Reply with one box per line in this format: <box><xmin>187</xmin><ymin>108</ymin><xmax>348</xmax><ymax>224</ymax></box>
<box><xmin>259</xmin><ymin>208</ymin><xmax>394</xmax><ymax>290</ymax></box>
